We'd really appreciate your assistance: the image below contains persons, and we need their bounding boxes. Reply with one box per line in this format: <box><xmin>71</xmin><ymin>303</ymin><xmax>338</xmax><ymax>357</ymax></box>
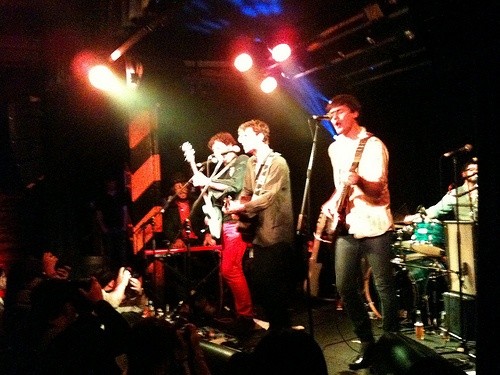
<box><xmin>0</xmin><ymin>119</ymin><xmax>478</xmax><ymax>375</ymax></box>
<box><xmin>321</xmin><ymin>95</ymin><xmax>401</xmax><ymax>368</ymax></box>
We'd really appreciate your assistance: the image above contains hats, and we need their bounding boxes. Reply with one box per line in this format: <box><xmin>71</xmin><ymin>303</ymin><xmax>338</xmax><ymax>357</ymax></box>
<box><xmin>169</xmin><ymin>172</ymin><xmax>190</xmax><ymax>188</ymax></box>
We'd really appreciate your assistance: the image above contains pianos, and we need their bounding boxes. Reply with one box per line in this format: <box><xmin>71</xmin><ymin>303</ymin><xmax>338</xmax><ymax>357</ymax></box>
<box><xmin>144</xmin><ymin>242</ymin><xmax>253</xmax><ymax>260</ymax></box>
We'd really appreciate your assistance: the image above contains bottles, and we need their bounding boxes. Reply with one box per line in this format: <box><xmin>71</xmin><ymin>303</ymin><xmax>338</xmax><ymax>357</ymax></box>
<box><xmin>145</xmin><ymin>300</ymin><xmax>165</xmax><ymax>321</ymax></box>
<box><xmin>415</xmin><ymin>310</ymin><xmax>425</xmax><ymax>340</ymax></box>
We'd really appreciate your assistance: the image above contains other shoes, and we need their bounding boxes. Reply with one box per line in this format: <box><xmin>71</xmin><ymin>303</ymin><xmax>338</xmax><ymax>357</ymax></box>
<box><xmin>349</xmin><ymin>355</ymin><xmax>369</xmax><ymax>369</ymax></box>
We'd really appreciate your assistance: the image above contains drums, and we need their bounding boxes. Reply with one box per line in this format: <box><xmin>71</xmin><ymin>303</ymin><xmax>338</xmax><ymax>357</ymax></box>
<box><xmin>364</xmin><ymin>252</ymin><xmax>451</xmax><ymax>321</ymax></box>
<box><xmin>394</xmin><ymin>229</ymin><xmax>415</xmax><ymax>252</ymax></box>
<box><xmin>410</xmin><ymin>220</ymin><xmax>446</xmax><ymax>257</ymax></box>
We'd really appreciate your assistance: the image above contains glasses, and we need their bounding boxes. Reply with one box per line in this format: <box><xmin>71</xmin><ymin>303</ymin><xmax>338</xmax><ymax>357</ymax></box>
<box><xmin>464</xmin><ymin>168</ymin><xmax>477</xmax><ymax>171</ymax></box>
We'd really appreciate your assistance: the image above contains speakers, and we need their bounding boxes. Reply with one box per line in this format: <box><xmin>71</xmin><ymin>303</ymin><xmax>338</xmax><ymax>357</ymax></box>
<box><xmin>370</xmin><ymin>331</ymin><xmax>467</xmax><ymax>375</ymax></box>
<box><xmin>143</xmin><ymin>244</ymin><xmax>222</xmax><ymax>314</ymax></box>
<box><xmin>199</xmin><ymin>340</ymin><xmax>243</xmax><ymax>375</ymax></box>
<box><xmin>443</xmin><ymin>220</ymin><xmax>477</xmax><ymax>296</ymax></box>
<box><xmin>443</xmin><ymin>292</ymin><xmax>477</xmax><ymax>343</ymax></box>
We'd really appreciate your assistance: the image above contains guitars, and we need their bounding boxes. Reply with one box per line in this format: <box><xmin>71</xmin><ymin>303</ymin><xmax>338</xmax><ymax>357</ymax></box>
<box><xmin>303</xmin><ymin>210</ymin><xmax>326</xmax><ymax>297</ymax></box>
<box><xmin>181</xmin><ymin>141</ymin><xmax>223</xmax><ymax>240</ymax></box>
<box><xmin>320</xmin><ymin>161</ymin><xmax>359</xmax><ymax>240</ymax></box>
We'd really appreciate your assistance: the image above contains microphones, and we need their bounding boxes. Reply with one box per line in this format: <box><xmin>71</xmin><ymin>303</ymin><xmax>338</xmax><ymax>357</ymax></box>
<box><xmin>463</xmin><ymin>262</ymin><xmax>468</xmax><ymax>276</ymax></box>
<box><xmin>443</xmin><ymin>145</ymin><xmax>472</xmax><ymax>158</ymax></box>
<box><xmin>311</xmin><ymin>115</ymin><xmax>332</xmax><ymax>120</ymax></box>
<box><xmin>197</xmin><ymin>158</ymin><xmax>218</xmax><ymax>169</ymax></box>
<box><xmin>209</xmin><ymin>145</ymin><xmax>241</xmax><ymax>159</ymax></box>
